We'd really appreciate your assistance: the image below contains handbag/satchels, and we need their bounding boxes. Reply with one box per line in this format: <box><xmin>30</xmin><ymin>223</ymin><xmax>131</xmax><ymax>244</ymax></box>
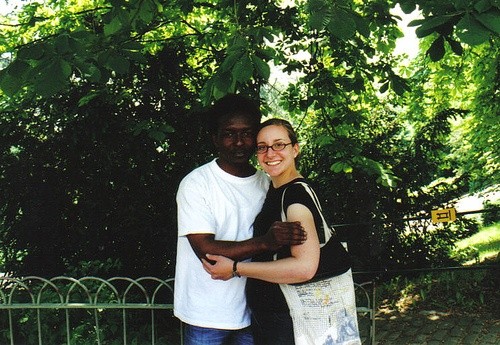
<box><xmin>273</xmin><ymin>182</ymin><xmax>362</xmax><ymax>345</ymax></box>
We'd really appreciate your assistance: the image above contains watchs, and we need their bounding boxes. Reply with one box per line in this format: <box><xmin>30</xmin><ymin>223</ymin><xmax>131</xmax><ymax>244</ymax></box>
<box><xmin>233</xmin><ymin>260</ymin><xmax>241</xmax><ymax>278</ymax></box>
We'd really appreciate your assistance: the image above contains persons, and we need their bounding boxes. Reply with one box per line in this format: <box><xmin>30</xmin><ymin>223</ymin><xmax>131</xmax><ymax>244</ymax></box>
<box><xmin>174</xmin><ymin>93</ymin><xmax>307</xmax><ymax>345</ymax></box>
<box><xmin>201</xmin><ymin>118</ymin><xmax>323</xmax><ymax>345</ymax></box>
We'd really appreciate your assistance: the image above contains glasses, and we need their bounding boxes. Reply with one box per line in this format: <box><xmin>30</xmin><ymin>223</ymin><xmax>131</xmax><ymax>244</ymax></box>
<box><xmin>255</xmin><ymin>142</ymin><xmax>292</xmax><ymax>154</ymax></box>
